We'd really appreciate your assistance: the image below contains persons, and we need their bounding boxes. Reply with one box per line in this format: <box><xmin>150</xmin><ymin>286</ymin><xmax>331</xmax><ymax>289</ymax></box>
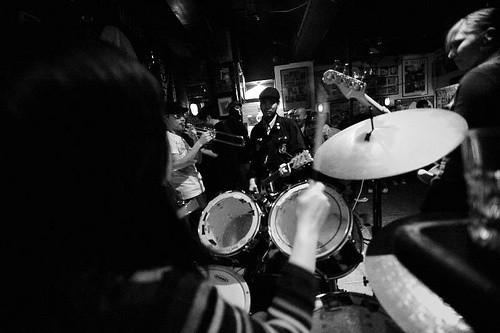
<box><xmin>200</xmin><ymin>107</ymin><xmax>237</xmax><ymax>202</ymax></box>
<box><xmin>0</xmin><ymin>0</ymin><xmax>330</xmax><ymax>333</ymax></box>
<box><xmin>162</xmin><ymin>101</ymin><xmax>215</xmax><ymax>211</ymax></box>
<box><xmin>336</xmin><ymin>102</ymin><xmax>406</xmax><ymax>202</ymax></box>
<box><xmin>431</xmin><ymin>9</ymin><xmax>500</xmax><ymax>208</ymax></box>
<box><xmin>288</xmin><ymin>109</ymin><xmax>296</xmax><ymax>121</ymax></box>
<box><xmin>250</xmin><ymin>87</ymin><xmax>306</xmax><ymax>193</ymax></box>
<box><xmin>416</xmin><ymin>100</ymin><xmax>428</xmax><ymax>109</ymax></box>
<box><xmin>215</xmin><ymin>101</ymin><xmax>248</xmax><ymax>142</ymax></box>
<box><xmin>294</xmin><ymin>107</ymin><xmax>314</xmax><ymax>156</ymax></box>
<box><xmin>311</xmin><ymin>111</ymin><xmax>317</xmax><ymax>121</ymax></box>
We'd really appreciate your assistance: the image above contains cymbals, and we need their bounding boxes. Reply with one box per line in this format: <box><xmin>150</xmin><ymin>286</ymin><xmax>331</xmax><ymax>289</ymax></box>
<box><xmin>312</xmin><ymin>108</ymin><xmax>468</xmax><ymax>181</ymax></box>
<box><xmin>365</xmin><ymin>209</ymin><xmax>500</xmax><ymax>333</ymax></box>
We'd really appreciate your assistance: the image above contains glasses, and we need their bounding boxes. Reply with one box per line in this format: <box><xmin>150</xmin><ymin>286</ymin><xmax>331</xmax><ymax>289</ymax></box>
<box><xmin>162</xmin><ymin>114</ymin><xmax>187</xmax><ymax>119</ymax></box>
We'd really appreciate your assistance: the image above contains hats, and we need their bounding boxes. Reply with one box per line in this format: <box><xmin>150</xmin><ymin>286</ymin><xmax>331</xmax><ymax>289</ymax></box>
<box><xmin>163</xmin><ymin>101</ymin><xmax>189</xmax><ymax>114</ymax></box>
<box><xmin>227</xmin><ymin>101</ymin><xmax>242</xmax><ymax>111</ymax></box>
<box><xmin>259</xmin><ymin>87</ymin><xmax>281</xmax><ymax>100</ymax></box>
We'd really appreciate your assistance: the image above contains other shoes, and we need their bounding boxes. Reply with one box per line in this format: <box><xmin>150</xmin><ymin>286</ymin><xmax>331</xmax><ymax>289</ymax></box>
<box><xmin>354</xmin><ymin>197</ymin><xmax>369</xmax><ymax>203</ymax></box>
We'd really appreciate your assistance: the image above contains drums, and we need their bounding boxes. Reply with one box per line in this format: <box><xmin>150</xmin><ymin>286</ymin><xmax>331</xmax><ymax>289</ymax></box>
<box><xmin>195</xmin><ymin>265</ymin><xmax>253</xmax><ymax>316</ymax></box>
<box><xmin>307</xmin><ymin>290</ymin><xmax>404</xmax><ymax>333</ymax></box>
<box><xmin>197</xmin><ymin>188</ymin><xmax>270</xmax><ymax>267</ymax></box>
<box><xmin>268</xmin><ymin>181</ymin><xmax>363</xmax><ymax>280</ymax></box>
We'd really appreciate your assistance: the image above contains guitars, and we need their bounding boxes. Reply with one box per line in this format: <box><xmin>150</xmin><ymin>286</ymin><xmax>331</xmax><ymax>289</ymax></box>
<box><xmin>321</xmin><ymin>60</ymin><xmax>391</xmax><ymax>115</ymax></box>
<box><xmin>238</xmin><ymin>149</ymin><xmax>314</xmax><ymax>217</ymax></box>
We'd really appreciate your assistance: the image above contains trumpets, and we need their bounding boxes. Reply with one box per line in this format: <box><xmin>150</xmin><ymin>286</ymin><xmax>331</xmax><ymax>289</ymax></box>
<box><xmin>183</xmin><ymin>123</ymin><xmax>245</xmax><ymax>147</ymax></box>
